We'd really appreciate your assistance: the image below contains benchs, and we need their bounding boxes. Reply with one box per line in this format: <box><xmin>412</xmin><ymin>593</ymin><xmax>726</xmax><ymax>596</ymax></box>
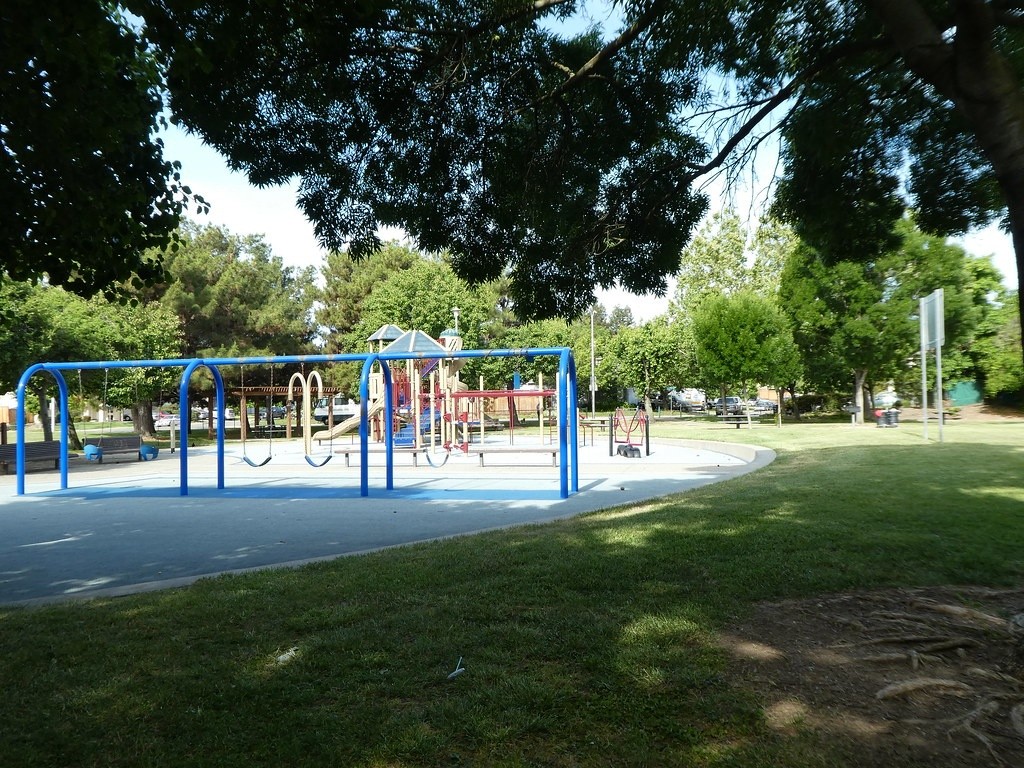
<box><xmin>717</xmin><ymin>421</ymin><xmax>762</xmax><ymax>429</ymax></box>
<box><xmin>82</xmin><ymin>435</ymin><xmax>143</xmax><ymax>464</ymax></box>
<box><xmin>469</xmin><ymin>449</ymin><xmax>560</xmax><ymax>468</ymax></box>
<box><xmin>587</xmin><ymin>424</ymin><xmax>610</xmax><ymax>431</ymax></box>
<box><xmin>335</xmin><ymin>449</ymin><xmax>426</xmax><ymax>467</ymax></box>
<box><xmin>252</xmin><ymin>430</ymin><xmax>294</xmax><ymax>438</ymax></box>
<box><xmin>0</xmin><ymin>440</ymin><xmax>60</xmax><ymax>474</ymax></box>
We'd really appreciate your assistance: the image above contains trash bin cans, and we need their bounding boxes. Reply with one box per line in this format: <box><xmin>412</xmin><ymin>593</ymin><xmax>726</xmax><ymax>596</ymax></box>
<box><xmin>876</xmin><ymin>408</ymin><xmax>900</xmax><ymax>429</ymax></box>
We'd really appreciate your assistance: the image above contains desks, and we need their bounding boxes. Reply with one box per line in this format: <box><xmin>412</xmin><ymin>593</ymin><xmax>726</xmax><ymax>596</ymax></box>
<box><xmin>717</xmin><ymin>415</ymin><xmax>757</xmax><ymax>429</ymax></box>
<box><xmin>590</xmin><ymin>419</ymin><xmax>610</xmax><ymax>431</ymax></box>
<box><xmin>256</xmin><ymin>424</ymin><xmax>289</xmax><ymax>437</ymax></box>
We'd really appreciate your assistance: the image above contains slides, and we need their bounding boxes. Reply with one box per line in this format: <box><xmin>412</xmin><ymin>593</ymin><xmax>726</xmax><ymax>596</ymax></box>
<box><xmin>313</xmin><ymin>389</ymin><xmax>386</xmax><ymax>441</ymax></box>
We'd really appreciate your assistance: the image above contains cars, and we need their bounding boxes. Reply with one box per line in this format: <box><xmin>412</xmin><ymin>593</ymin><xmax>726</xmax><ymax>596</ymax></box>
<box><xmin>194</xmin><ymin>408</ymin><xmax>236</xmax><ymax>419</ymax></box>
<box><xmin>715</xmin><ymin>397</ymin><xmax>742</xmax><ymax>415</ymax></box>
<box><xmin>123</xmin><ymin>411</ymin><xmax>180</xmax><ymax>425</ymax></box>
<box><xmin>260</xmin><ymin>406</ymin><xmax>285</xmax><ymax>419</ymax></box>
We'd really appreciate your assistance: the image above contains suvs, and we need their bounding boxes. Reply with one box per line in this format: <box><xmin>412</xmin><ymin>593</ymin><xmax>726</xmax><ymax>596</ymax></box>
<box><xmin>313</xmin><ymin>396</ymin><xmax>357</xmax><ymax>425</ymax></box>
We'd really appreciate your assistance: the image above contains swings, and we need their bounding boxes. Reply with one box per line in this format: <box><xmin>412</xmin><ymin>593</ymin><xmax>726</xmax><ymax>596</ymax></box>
<box><xmin>240</xmin><ymin>364</ymin><xmax>275</xmax><ymax>467</ymax></box>
<box><xmin>78</xmin><ymin>369</ymin><xmax>113</xmax><ymax>461</ymax></box>
<box><xmin>301</xmin><ymin>362</ymin><xmax>334</xmax><ymax>468</ymax></box>
<box><xmin>133</xmin><ymin>366</ymin><xmax>165</xmax><ymax>461</ymax></box>
<box><xmin>418</xmin><ymin>359</ymin><xmax>454</xmax><ymax>468</ymax></box>
